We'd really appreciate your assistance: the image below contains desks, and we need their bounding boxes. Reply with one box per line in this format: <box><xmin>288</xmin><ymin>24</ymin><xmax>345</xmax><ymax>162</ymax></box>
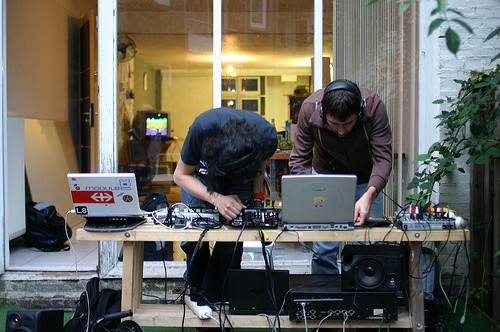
<box><xmin>75</xmin><ymin>216</ymin><xmax>471</xmax><ymax>332</ymax></box>
<box><xmin>129</xmin><ymin>136</ymin><xmax>185</xmax><ymax>186</ymax></box>
<box><xmin>268</xmin><ymin>149</ymin><xmax>292</xmax><ymax>201</ymax></box>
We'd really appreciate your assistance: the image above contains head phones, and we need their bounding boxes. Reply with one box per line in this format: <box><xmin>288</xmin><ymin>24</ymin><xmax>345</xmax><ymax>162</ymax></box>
<box><xmin>320</xmin><ymin>81</ymin><xmax>367</xmax><ymax>121</ymax></box>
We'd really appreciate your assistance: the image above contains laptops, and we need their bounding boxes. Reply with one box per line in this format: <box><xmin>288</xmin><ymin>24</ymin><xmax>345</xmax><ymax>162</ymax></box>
<box><xmin>281</xmin><ymin>175</ymin><xmax>357</xmax><ymax>231</ymax></box>
<box><xmin>65</xmin><ymin>173</ymin><xmax>152</xmax><ymax>217</ymax></box>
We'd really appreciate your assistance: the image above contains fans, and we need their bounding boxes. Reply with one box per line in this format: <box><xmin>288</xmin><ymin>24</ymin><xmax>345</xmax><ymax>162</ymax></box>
<box><xmin>117</xmin><ymin>36</ymin><xmax>138</xmax><ymax>63</ymax></box>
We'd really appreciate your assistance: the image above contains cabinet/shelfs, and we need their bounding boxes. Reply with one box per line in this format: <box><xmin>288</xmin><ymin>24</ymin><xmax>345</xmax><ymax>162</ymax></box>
<box><xmin>283</xmin><ymin>93</ymin><xmax>312</xmax><ymax>125</ymax></box>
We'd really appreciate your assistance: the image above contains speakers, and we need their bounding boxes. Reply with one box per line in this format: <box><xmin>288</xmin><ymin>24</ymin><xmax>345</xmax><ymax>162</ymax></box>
<box><xmin>6</xmin><ymin>310</ymin><xmax>64</xmax><ymax>332</ymax></box>
<box><xmin>340</xmin><ymin>243</ymin><xmax>409</xmax><ymax>293</ymax></box>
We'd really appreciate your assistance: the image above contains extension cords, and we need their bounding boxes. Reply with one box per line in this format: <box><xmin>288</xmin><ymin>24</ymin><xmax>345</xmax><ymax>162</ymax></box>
<box><xmin>185</xmin><ymin>293</ymin><xmax>215</xmax><ymax>320</ymax></box>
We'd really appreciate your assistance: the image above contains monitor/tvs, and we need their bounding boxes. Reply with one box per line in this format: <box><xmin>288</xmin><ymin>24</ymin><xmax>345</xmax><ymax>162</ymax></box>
<box><xmin>138</xmin><ymin>109</ymin><xmax>171</xmax><ymax>141</ymax></box>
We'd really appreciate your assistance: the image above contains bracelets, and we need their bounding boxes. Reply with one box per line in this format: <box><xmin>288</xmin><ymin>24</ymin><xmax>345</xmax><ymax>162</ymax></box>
<box><xmin>214</xmin><ymin>194</ymin><xmax>223</xmax><ymax>210</ymax></box>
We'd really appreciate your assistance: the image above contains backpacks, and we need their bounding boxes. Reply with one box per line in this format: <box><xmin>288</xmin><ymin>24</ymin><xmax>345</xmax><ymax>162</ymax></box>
<box><xmin>24</xmin><ymin>201</ymin><xmax>72</xmax><ymax>251</ymax></box>
<box><xmin>118</xmin><ymin>192</ymin><xmax>169</xmax><ymax>261</ymax></box>
<box><xmin>63</xmin><ymin>277</ymin><xmax>122</xmax><ymax>332</ymax></box>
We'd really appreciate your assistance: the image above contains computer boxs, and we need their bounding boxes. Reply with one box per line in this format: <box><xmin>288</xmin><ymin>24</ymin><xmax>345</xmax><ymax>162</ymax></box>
<box><xmin>287</xmin><ymin>274</ymin><xmax>399</xmax><ymax>322</ymax></box>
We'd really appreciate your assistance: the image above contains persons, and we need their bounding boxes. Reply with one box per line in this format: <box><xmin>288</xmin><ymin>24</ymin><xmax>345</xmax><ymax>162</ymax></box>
<box><xmin>173</xmin><ymin>108</ymin><xmax>279</xmax><ymax>282</ymax></box>
<box><xmin>288</xmin><ymin>77</ymin><xmax>392</xmax><ymax>286</ymax></box>
